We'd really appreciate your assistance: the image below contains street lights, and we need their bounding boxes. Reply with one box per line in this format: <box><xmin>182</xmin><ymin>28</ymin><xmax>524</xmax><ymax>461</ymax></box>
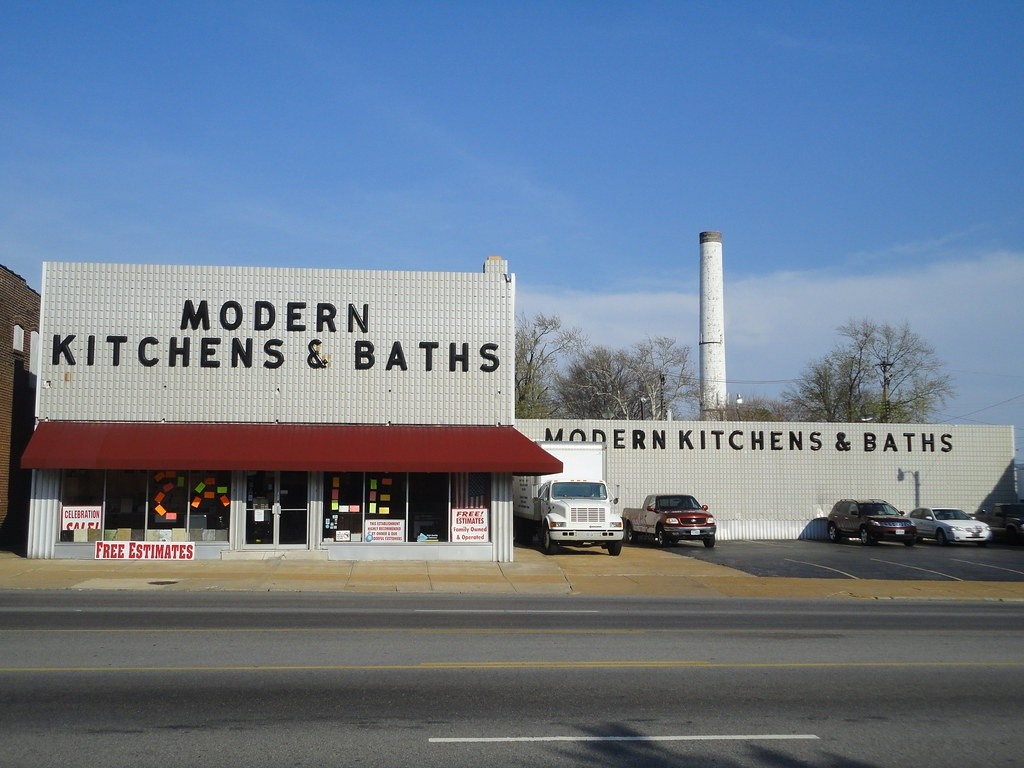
<box><xmin>735</xmin><ymin>393</ymin><xmax>744</xmax><ymax>420</ymax></box>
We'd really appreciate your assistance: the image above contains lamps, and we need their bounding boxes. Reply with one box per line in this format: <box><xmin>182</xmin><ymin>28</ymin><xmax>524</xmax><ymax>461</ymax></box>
<box><xmin>735</xmin><ymin>393</ymin><xmax>743</xmax><ymax>421</ymax></box>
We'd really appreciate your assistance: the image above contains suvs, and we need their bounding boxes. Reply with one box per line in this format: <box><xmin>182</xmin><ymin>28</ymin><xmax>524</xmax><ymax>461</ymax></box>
<box><xmin>969</xmin><ymin>501</ymin><xmax>1024</xmax><ymax>538</ymax></box>
<box><xmin>827</xmin><ymin>498</ymin><xmax>917</xmax><ymax>546</ymax></box>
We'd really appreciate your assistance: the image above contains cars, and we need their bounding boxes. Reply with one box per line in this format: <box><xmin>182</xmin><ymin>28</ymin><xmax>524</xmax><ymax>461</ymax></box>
<box><xmin>904</xmin><ymin>507</ymin><xmax>992</xmax><ymax>544</ymax></box>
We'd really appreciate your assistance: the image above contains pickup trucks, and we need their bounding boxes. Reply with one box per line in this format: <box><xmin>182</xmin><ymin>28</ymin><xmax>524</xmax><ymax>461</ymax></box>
<box><xmin>624</xmin><ymin>494</ymin><xmax>716</xmax><ymax>549</ymax></box>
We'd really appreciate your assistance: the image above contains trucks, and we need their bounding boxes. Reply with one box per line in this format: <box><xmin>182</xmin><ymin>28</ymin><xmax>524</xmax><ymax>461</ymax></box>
<box><xmin>520</xmin><ymin>443</ymin><xmax>623</xmax><ymax>553</ymax></box>
